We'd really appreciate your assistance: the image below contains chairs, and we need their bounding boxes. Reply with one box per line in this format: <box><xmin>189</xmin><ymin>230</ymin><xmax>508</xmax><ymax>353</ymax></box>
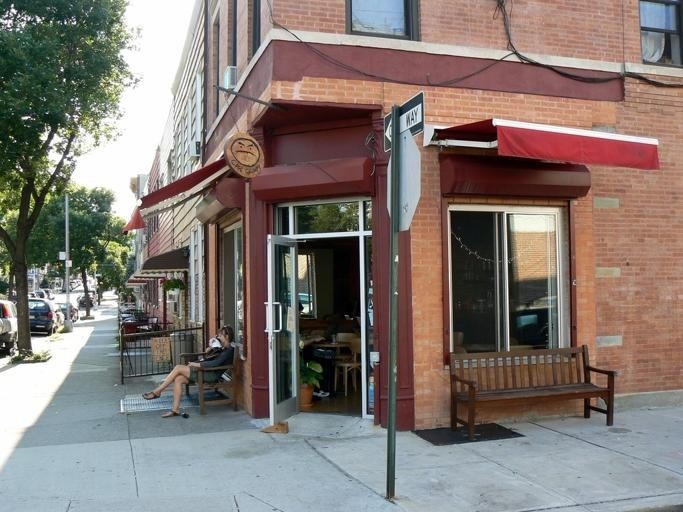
<box><xmin>332</xmin><ymin>332</ymin><xmax>362</xmax><ymax>397</ymax></box>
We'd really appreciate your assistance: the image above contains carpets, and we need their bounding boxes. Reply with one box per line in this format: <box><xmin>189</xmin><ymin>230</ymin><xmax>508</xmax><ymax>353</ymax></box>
<box><xmin>408</xmin><ymin>422</ymin><xmax>528</xmax><ymax>446</ymax></box>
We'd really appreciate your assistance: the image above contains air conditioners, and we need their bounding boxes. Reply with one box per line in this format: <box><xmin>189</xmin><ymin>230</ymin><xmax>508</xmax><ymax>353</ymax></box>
<box><xmin>184</xmin><ymin>140</ymin><xmax>200</xmax><ymax>161</ymax></box>
<box><xmin>223</xmin><ymin>65</ymin><xmax>237</xmax><ymax>90</ymax></box>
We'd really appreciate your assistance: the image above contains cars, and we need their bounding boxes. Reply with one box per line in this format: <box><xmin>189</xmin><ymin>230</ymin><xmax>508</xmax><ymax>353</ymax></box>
<box><xmin>28</xmin><ymin>282</ymin><xmax>98</xmax><ymax>336</ymax></box>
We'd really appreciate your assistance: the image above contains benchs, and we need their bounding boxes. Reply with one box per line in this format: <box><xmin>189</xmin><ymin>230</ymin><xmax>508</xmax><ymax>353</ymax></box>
<box><xmin>448</xmin><ymin>344</ymin><xmax>616</xmax><ymax>441</ymax></box>
<box><xmin>177</xmin><ymin>343</ymin><xmax>240</xmax><ymax>415</ymax></box>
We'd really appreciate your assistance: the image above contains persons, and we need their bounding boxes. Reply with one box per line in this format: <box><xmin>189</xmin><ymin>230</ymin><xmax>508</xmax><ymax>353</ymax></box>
<box><xmin>142</xmin><ymin>325</ymin><xmax>235</xmax><ymax>418</ymax></box>
<box><xmin>276</xmin><ymin>300</ymin><xmax>331</xmax><ymax>398</ymax></box>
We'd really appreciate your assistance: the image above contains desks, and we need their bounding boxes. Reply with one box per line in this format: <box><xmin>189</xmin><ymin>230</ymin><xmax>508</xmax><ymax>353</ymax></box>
<box><xmin>313</xmin><ymin>342</ymin><xmax>349</xmax><ymax>394</ymax></box>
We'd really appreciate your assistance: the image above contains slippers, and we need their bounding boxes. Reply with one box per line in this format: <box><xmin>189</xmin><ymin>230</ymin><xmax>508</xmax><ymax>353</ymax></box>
<box><xmin>162</xmin><ymin>410</ymin><xmax>179</xmax><ymax>417</ymax></box>
<box><xmin>142</xmin><ymin>392</ymin><xmax>160</xmax><ymax>400</ymax></box>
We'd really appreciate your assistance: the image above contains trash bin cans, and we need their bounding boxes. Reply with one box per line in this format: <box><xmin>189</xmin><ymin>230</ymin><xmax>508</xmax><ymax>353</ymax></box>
<box><xmin>170</xmin><ymin>333</ymin><xmax>194</xmax><ymax>368</ymax></box>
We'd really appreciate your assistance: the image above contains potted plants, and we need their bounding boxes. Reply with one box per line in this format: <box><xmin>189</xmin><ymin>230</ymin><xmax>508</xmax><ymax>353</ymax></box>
<box><xmin>162</xmin><ymin>277</ymin><xmax>183</xmax><ymax>302</ymax></box>
<box><xmin>298</xmin><ymin>356</ymin><xmax>323</xmax><ymax>407</ymax></box>
